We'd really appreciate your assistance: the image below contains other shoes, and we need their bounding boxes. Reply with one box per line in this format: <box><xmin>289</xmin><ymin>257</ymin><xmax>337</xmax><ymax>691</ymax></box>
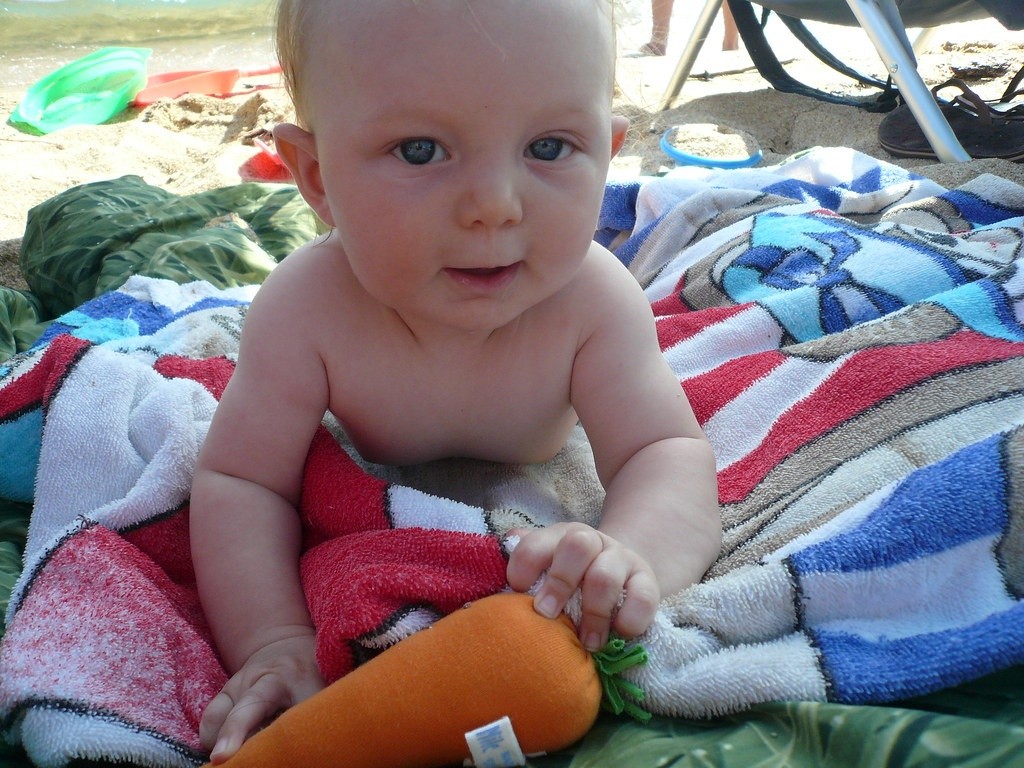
<box><xmin>877</xmin><ymin>79</ymin><xmax>1024</xmax><ymax>160</ymax></box>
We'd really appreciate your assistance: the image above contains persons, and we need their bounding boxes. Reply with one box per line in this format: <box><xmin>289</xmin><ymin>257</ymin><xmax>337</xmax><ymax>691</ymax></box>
<box><xmin>188</xmin><ymin>0</ymin><xmax>722</xmax><ymax>768</ymax></box>
<box><xmin>626</xmin><ymin>0</ymin><xmax>739</xmax><ymax>56</ymax></box>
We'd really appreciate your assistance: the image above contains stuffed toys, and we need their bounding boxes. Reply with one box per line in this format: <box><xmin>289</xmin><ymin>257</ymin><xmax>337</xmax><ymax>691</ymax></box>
<box><xmin>202</xmin><ymin>593</ymin><xmax>652</xmax><ymax>768</ymax></box>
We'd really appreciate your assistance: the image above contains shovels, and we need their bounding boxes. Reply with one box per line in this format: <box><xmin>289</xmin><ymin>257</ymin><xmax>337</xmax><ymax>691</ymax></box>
<box><xmin>135</xmin><ymin>63</ymin><xmax>284</xmax><ymax>107</ymax></box>
